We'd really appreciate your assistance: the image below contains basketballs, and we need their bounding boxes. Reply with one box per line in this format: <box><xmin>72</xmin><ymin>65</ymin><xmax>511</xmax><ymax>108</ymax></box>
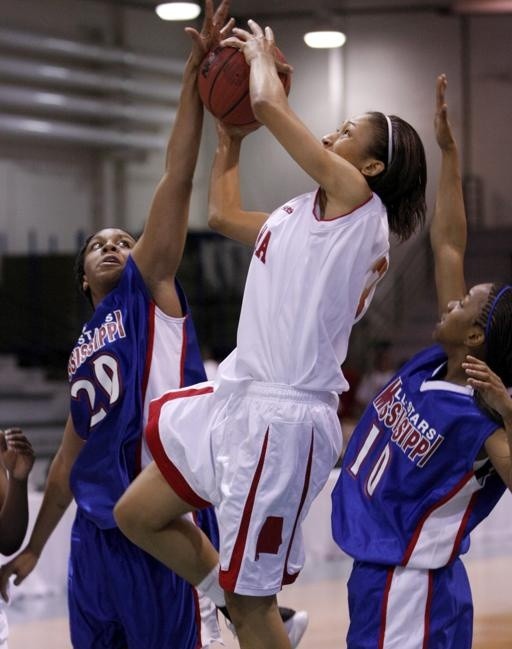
<box><xmin>198</xmin><ymin>34</ymin><xmax>291</xmax><ymax>125</ymax></box>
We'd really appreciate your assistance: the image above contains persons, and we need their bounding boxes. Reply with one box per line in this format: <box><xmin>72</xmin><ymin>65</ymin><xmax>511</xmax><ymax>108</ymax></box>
<box><xmin>0</xmin><ymin>426</ymin><xmax>36</xmax><ymax>557</ymax></box>
<box><xmin>331</xmin><ymin>72</ymin><xmax>512</xmax><ymax>649</ymax></box>
<box><xmin>112</xmin><ymin>18</ymin><xmax>427</xmax><ymax>649</ymax></box>
<box><xmin>1</xmin><ymin>1</ymin><xmax>237</xmax><ymax>649</ymax></box>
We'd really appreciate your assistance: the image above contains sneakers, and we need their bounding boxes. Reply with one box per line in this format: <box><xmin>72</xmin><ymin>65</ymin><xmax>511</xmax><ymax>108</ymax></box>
<box><xmin>228</xmin><ymin>606</ymin><xmax>310</xmax><ymax>648</ymax></box>
